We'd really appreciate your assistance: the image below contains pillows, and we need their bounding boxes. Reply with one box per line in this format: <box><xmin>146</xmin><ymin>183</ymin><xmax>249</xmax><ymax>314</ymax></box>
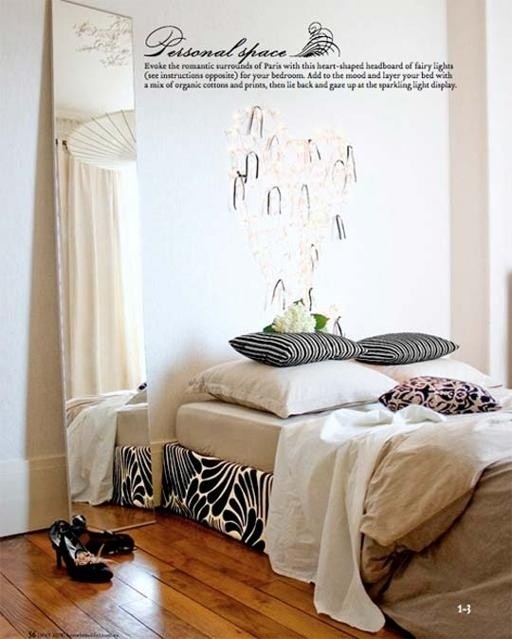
<box><xmin>120</xmin><ymin>381</ymin><xmax>148</xmax><ymax>405</ymax></box>
<box><xmin>187</xmin><ymin>331</ymin><xmax>503</xmax><ymax>422</ymax></box>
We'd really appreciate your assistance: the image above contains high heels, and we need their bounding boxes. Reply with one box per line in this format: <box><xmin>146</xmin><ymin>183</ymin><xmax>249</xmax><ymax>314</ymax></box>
<box><xmin>48</xmin><ymin>520</ymin><xmax>113</xmax><ymax>583</ymax></box>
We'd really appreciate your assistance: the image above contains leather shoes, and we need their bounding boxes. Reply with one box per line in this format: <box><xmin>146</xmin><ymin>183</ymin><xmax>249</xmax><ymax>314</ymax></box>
<box><xmin>71</xmin><ymin>516</ymin><xmax>134</xmax><ymax>556</ymax></box>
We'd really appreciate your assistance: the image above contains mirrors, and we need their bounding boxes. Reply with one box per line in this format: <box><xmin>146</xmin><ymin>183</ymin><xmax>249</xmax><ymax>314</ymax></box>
<box><xmin>51</xmin><ymin>0</ymin><xmax>158</xmax><ymax>532</ymax></box>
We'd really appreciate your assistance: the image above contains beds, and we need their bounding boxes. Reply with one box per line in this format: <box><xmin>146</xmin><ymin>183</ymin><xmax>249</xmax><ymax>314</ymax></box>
<box><xmin>160</xmin><ymin>379</ymin><xmax>510</xmax><ymax>639</ymax></box>
<box><xmin>65</xmin><ymin>390</ymin><xmax>157</xmax><ymax>511</ymax></box>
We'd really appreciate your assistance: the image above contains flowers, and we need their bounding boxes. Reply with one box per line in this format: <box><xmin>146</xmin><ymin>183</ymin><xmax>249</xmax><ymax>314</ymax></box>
<box><xmin>257</xmin><ymin>306</ymin><xmax>330</xmax><ymax>341</ymax></box>
